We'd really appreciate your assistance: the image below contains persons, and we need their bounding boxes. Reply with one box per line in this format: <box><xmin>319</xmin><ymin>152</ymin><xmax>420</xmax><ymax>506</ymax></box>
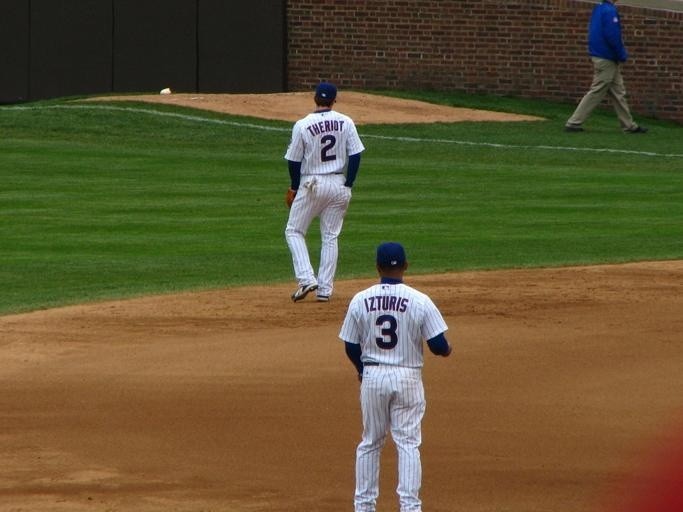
<box><xmin>560</xmin><ymin>0</ymin><xmax>651</xmax><ymax>137</ymax></box>
<box><xmin>336</xmin><ymin>240</ymin><xmax>453</xmax><ymax>512</ymax></box>
<box><xmin>280</xmin><ymin>81</ymin><xmax>366</xmax><ymax>305</ymax></box>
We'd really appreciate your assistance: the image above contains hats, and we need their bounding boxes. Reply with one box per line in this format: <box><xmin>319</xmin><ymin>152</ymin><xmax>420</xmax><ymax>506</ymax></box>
<box><xmin>376</xmin><ymin>242</ymin><xmax>406</xmax><ymax>269</ymax></box>
<box><xmin>315</xmin><ymin>81</ymin><xmax>338</xmax><ymax>102</ymax></box>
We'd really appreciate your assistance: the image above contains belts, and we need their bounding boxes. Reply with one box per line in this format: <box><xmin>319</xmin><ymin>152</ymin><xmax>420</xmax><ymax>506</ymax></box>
<box><xmin>362</xmin><ymin>361</ymin><xmax>381</xmax><ymax>367</ymax></box>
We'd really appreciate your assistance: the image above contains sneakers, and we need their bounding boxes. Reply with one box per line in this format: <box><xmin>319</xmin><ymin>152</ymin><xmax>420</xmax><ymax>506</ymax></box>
<box><xmin>290</xmin><ymin>280</ymin><xmax>318</xmax><ymax>303</ymax></box>
<box><xmin>564</xmin><ymin>121</ymin><xmax>585</xmax><ymax>130</ymax></box>
<box><xmin>315</xmin><ymin>293</ymin><xmax>331</xmax><ymax>302</ymax></box>
<box><xmin>623</xmin><ymin>124</ymin><xmax>649</xmax><ymax>134</ymax></box>
